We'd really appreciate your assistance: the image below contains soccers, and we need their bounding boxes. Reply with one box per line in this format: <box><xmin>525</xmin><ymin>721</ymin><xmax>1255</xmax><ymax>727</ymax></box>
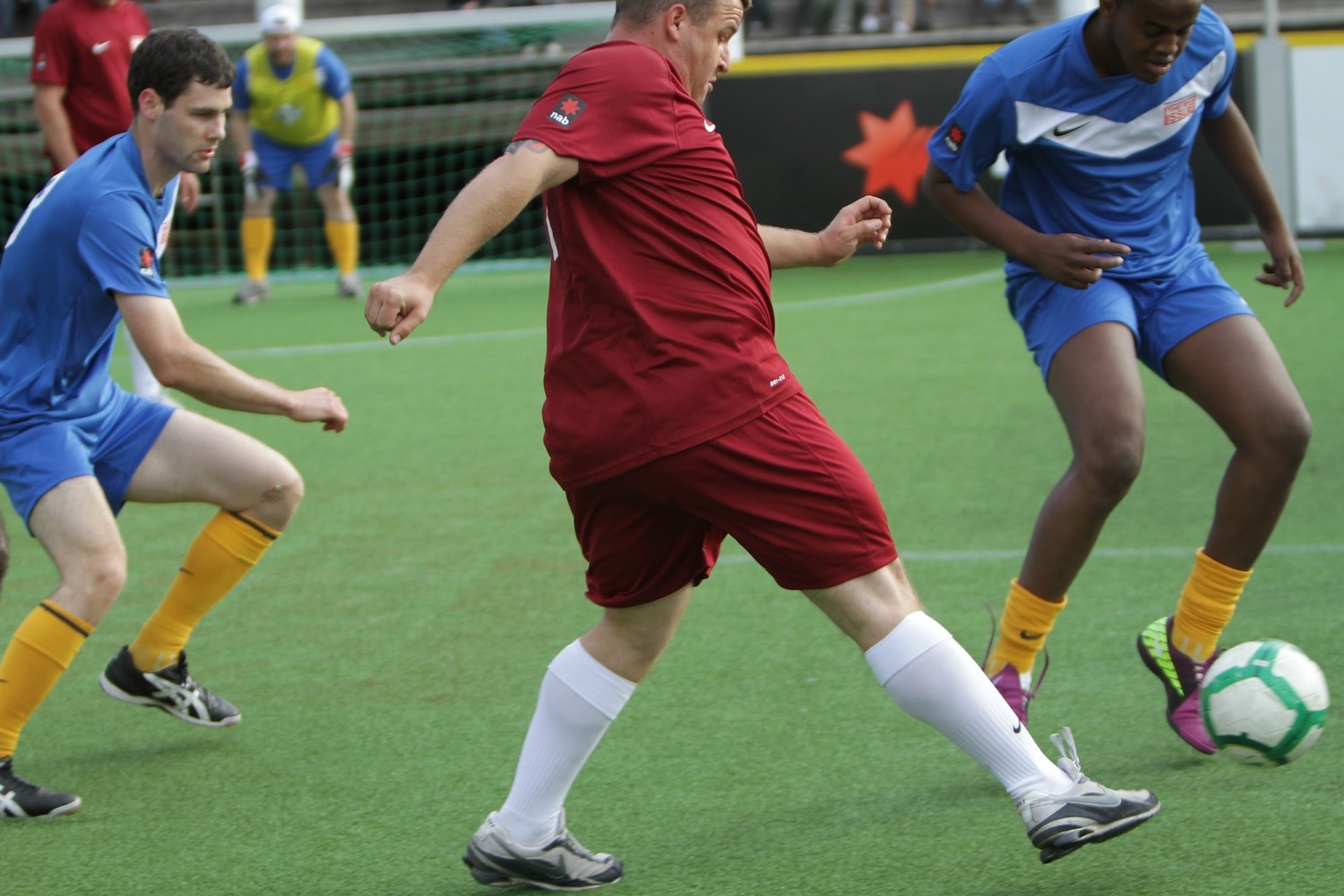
<box><xmin>1198</xmin><ymin>641</ymin><xmax>1328</xmax><ymax>765</ymax></box>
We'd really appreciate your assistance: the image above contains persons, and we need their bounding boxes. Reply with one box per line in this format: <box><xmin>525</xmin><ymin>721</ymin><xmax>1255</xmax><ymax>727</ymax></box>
<box><xmin>229</xmin><ymin>4</ymin><xmax>367</xmax><ymax>308</ymax></box>
<box><xmin>358</xmin><ymin>0</ymin><xmax>1160</xmax><ymax>892</ymax></box>
<box><xmin>0</xmin><ymin>22</ymin><xmax>352</xmax><ymax>824</ymax></box>
<box><xmin>919</xmin><ymin>1</ymin><xmax>1315</xmax><ymax>756</ymax></box>
<box><xmin>32</xmin><ymin>0</ymin><xmax>201</xmax><ymax>410</ymax></box>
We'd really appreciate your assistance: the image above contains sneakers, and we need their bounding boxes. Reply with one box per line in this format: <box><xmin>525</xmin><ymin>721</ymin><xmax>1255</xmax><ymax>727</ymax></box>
<box><xmin>0</xmin><ymin>757</ymin><xmax>82</xmax><ymax>819</ymax></box>
<box><xmin>1136</xmin><ymin>615</ymin><xmax>1218</xmax><ymax>754</ymax></box>
<box><xmin>1015</xmin><ymin>727</ymin><xmax>1162</xmax><ymax>864</ymax></box>
<box><xmin>462</xmin><ymin>812</ymin><xmax>624</xmax><ymax>891</ymax></box>
<box><xmin>98</xmin><ymin>645</ymin><xmax>240</xmax><ymax>726</ymax></box>
<box><xmin>978</xmin><ymin>603</ymin><xmax>1049</xmax><ymax>727</ymax></box>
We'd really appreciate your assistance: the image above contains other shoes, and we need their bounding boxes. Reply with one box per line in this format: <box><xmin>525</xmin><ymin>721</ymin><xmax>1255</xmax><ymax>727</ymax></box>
<box><xmin>337</xmin><ymin>276</ymin><xmax>358</xmax><ymax>297</ymax></box>
<box><xmin>231</xmin><ymin>281</ymin><xmax>268</xmax><ymax>305</ymax></box>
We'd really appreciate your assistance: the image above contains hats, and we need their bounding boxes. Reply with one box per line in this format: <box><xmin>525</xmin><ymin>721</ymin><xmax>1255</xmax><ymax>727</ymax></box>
<box><xmin>261</xmin><ymin>7</ymin><xmax>300</xmax><ymax>35</ymax></box>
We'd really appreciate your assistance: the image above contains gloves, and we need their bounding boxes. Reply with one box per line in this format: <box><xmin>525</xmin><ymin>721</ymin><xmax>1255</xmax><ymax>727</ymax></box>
<box><xmin>239</xmin><ymin>152</ymin><xmax>258</xmax><ymax>201</ymax></box>
<box><xmin>336</xmin><ymin>147</ymin><xmax>354</xmax><ymax>189</ymax></box>
